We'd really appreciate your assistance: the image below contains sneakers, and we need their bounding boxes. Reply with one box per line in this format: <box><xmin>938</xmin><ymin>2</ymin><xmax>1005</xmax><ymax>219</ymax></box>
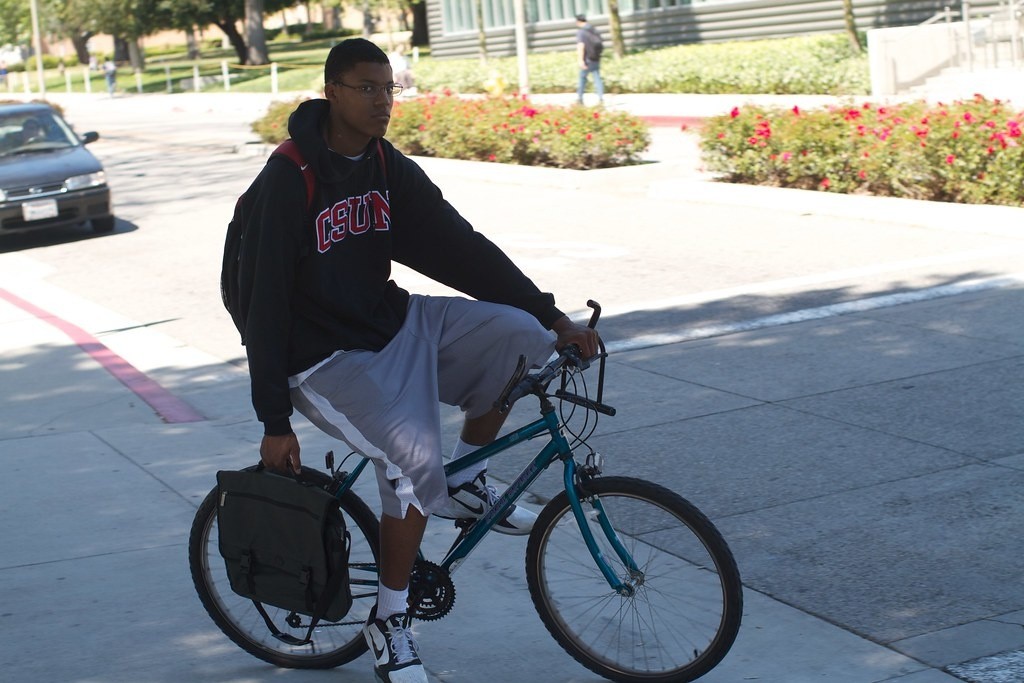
<box><xmin>429</xmin><ymin>468</ymin><xmax>539</xmax><ymax>536</ymax></box>
<box><xmin>362</xmin><ymin>604</ymin><xmax>428</xmax><ymax>683</ymax></box>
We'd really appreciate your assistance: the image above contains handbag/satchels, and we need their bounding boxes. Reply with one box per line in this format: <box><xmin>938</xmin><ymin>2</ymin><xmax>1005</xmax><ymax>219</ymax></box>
<box><xmin>217</xmin><ymin>453</ymin><xmax>352</xmax><ymax>648</ymax></box>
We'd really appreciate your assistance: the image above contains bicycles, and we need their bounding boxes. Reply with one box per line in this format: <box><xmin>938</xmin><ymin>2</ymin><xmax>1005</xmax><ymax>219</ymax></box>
<box><xmin>185</xmin><ymin>299</ymin><xmax>743</xmax><ymax>683</ymax></box>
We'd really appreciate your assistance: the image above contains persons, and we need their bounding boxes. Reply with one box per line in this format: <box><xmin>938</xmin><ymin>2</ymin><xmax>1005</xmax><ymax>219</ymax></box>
<box><xmin>387</xmin><ymin>43</ymin><xmax>416</xmax><ymax>96</ymax></box>
<box><xmin>23</xmin><ymin>120</ymin><xmax>41</xmax><ymax>144</ymax></box>
<box><xmin>574</xmin><ymin>13</ymin><xmax>605</xmax><ymax>109</ymax></box>
<box><xmin>221</xmin><ymin>39</ymin><xmax>600</xmax><ymax>683</ymax></box>
<box><xmin>101</xmin><ymin>56</ymin><xmax>116</xmax><ymax>97</ymax></box>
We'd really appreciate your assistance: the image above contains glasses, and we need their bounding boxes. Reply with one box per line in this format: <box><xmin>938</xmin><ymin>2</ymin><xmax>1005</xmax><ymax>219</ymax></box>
<box><xmin>335</xmin><ymin>81</ymin><xmax>403</xmax><ymax>97</ymax></box>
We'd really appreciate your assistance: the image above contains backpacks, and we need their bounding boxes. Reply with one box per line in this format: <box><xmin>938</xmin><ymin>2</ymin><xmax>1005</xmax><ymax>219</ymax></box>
<box><xmin>583</xmin><ymin>28</ymin><xmax>602</xmax><ymax>61</ymax></box>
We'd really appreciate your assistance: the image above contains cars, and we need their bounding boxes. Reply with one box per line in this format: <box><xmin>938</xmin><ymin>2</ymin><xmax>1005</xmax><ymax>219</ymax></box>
<box><xmin>0</xmin><ymin>105</ymin><xmax>116</xmax><ymax>235</ymax></box>
